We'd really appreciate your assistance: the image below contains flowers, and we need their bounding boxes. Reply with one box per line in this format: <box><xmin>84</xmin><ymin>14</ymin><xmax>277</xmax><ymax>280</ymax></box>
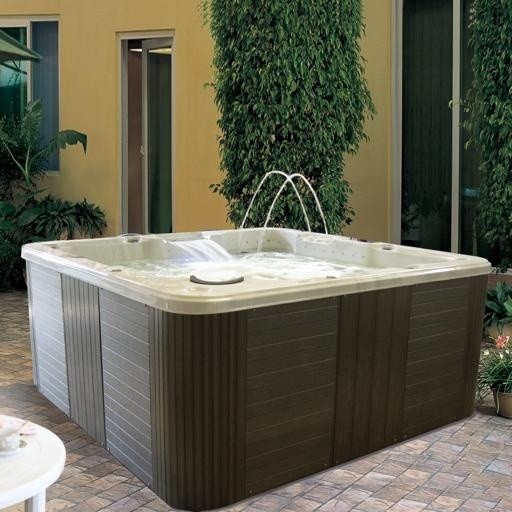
<box><xmin>475</xmin><ymin>331</ymin><xmax>512</xmax><ymax>395</ymax></box>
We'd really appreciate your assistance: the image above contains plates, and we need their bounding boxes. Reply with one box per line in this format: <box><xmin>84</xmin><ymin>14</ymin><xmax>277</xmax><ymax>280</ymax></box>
<box><xmin>0</xmin><ymin>439</ymin><xmax>31</xmax><ymax>456</ymax></box>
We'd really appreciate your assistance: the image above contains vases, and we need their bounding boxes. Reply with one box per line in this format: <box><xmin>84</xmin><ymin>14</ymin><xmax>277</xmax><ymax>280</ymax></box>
<box><xmin>491</xmin><ymin>390</ymin><xmax>512</xmax><ymax>418</ymax></box>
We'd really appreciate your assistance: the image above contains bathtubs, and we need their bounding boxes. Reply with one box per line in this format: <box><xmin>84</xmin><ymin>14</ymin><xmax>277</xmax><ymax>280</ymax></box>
<box><xmin>18</xmin><ymin>225</ymin><xmax>493</xmax><ymax>512</ymax></box>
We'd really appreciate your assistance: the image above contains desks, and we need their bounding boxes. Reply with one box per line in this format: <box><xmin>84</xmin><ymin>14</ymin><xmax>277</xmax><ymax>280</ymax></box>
<box><xmin>0</xmin><ymin>415</ymin><xmax>66</xmax><ymax>512</ymax></box>
